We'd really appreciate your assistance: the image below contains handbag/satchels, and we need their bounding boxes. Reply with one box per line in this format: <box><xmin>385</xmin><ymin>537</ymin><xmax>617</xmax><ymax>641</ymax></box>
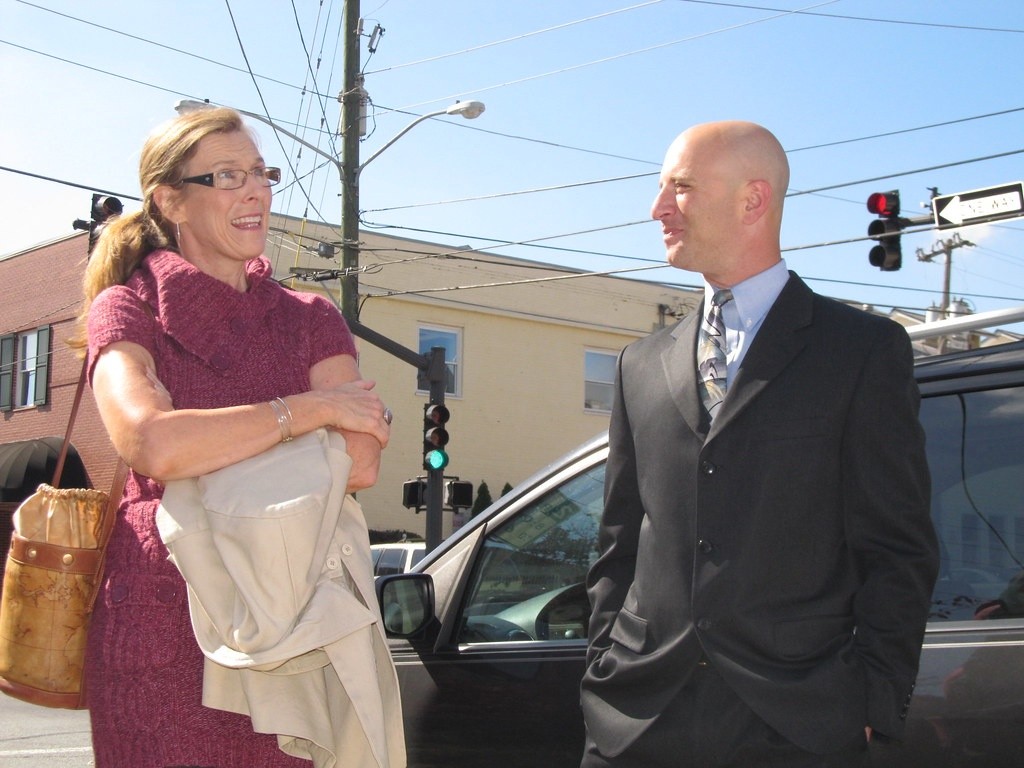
<box><xmin>0</xmin><ymin>292</ymin><xmax>163</xmax><ymax>710</ymax></box>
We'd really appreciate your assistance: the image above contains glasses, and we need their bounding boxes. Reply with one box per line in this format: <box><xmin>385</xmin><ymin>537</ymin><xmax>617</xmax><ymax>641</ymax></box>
<box><xmin>176</xmin><ymin>166</ymin><xmax>282</xmax><ymax>191</ymax></box>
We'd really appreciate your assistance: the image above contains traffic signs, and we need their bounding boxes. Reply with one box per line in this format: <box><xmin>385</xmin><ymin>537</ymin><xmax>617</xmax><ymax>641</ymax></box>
<box><xmin>931</xmin><ymin>180</ymin><xmax>1023</xmax><ymax>230</ymax></box>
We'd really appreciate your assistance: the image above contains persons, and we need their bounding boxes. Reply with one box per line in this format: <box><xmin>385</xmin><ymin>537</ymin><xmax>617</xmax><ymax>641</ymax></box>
<box><xmin>83</xmin><ymin>106</ymin><xmax>393</xmax><ymax>768</ymax></box>
<box><xmin>576</xmin><ymin>121</ymin><xmax>942</xmax><ymax>768</ymax></box>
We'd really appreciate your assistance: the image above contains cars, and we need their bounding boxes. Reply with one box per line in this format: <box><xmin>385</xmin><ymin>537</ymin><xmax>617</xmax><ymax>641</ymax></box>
<box><xmin>370</xmin><ymin>539</ymin><xmax>428</xmax><ymax>584</ymax></box>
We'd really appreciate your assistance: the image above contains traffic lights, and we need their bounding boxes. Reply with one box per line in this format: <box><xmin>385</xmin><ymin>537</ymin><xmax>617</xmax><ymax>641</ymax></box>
<box><xmin>402</xmin><ymin>478</ymin><xmax>426</xmax><ymax>510</ymax></box>
<box><xmin>446</xmin><ymin>482</ymin><xmax>473</xmax><ymax>508</ymax></box>
<box><xmin>865</xmin><ymin>189</ymin><xmax>904</xmax><ymax>273</ymax></box>
<box><xmin>89</xmin><ymin>194</ymin><xmax>123</xmax><ymax>266</ymax></box>
<box><xmin>420</xmin><ymin>401</ymin><xmax>453</xmax><ymax>470</ymax></box>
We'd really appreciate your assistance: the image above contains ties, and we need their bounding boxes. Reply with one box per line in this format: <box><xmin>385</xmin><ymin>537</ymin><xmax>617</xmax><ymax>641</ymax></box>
<box><xmin>697</xmin><ymin>288</ymin><xmax>733</xmax><ymax>429</ymax></box>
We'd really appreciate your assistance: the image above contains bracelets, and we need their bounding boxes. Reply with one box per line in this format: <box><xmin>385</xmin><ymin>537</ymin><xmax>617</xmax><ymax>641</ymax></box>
<box><xmin>268</xmin><ymin>397</ymin><xmax>293</xmax><ymax>443</ymax></box>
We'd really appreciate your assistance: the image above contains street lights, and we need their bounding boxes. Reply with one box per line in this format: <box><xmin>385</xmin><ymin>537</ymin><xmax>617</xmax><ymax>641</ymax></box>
<box><xmin>339</xmin><ymin>100</ymin><xmax>487</xmax><ymax>502</ymax></box>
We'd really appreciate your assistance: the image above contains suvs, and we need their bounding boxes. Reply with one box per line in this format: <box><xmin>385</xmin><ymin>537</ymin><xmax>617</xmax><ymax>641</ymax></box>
<box><xmin>377</xmin><ymin>310</ymin><xmax>1024</xmax><ymax>768</ymax></box>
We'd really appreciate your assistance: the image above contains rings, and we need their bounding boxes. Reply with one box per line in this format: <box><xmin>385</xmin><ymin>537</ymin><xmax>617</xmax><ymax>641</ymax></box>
<box><xmin>382</xmin><ymin>408</ymin><xmax>393</xmax><ymax>427</ymax></box>
